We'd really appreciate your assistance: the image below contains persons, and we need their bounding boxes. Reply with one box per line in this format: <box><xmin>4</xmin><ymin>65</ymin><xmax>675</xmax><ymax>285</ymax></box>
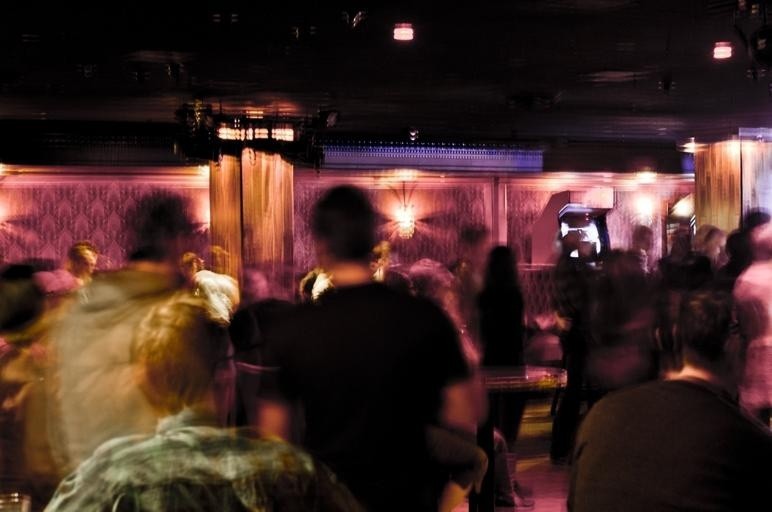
<box><xmin>1</xmin><ymin>185</ymin><xmax>527</xmax><ymax>512</ymax></box>
<box><xmin>550</xmin><ymin>214</ymin><xmax>770</xmax><ymax>512</ymax></box>
